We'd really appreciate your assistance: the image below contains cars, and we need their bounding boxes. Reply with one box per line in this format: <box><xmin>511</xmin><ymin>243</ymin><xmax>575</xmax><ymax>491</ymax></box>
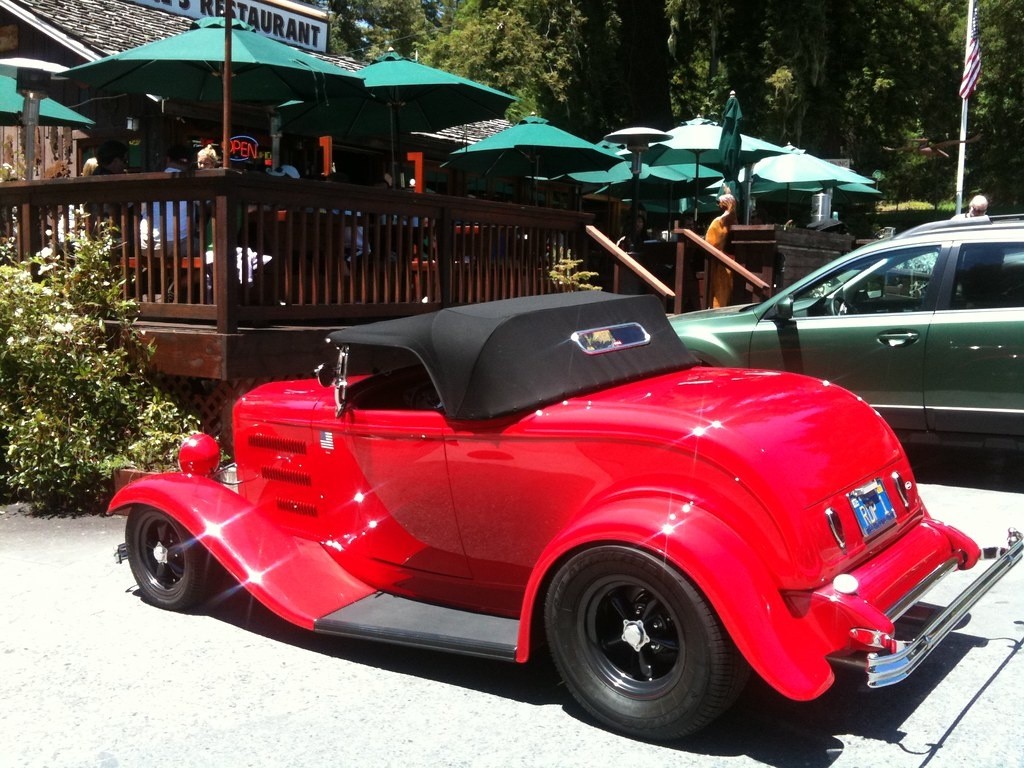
<box><xmin>105</xmin><ymin>289</ymin><xmax>1024</xmax><ymax>742</ymax></box>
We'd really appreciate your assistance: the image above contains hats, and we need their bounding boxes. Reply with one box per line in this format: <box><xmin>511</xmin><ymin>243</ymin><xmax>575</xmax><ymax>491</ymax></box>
<box><xmin>166</xmin><ymin>144</ymin><xmax>193</xmax><ymax>164</ymax></box>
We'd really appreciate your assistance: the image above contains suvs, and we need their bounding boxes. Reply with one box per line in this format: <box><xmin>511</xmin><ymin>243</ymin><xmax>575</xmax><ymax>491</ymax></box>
<box><xmin>665</xmin><ymin>212</ymin><xmax>1024</xmax><ymax>456</ymax></box>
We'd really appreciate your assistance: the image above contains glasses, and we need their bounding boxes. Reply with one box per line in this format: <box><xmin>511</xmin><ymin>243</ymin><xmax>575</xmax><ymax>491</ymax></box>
<box><xmin>119</xmin><ymin>157</ymin><xmax>129</xmax><ymax>163</ymax></box>
<box><xmin>973</xmin><ymin>207</ymin><xmax>987</xmax><ymax>213</ymax></box>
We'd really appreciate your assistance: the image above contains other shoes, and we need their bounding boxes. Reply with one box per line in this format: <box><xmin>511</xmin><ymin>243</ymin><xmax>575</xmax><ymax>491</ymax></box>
<box><xmin>130</xmin><ymin>267</ymin><xmax>147</xmax><ymax>285</ymax></box>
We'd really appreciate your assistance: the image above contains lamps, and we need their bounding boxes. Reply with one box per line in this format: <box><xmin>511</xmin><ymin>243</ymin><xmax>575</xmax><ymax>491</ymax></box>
<box><xmin>126</xmin><ymin>113</ymin><xmax>142</xmax><ymax>131</ymax></box>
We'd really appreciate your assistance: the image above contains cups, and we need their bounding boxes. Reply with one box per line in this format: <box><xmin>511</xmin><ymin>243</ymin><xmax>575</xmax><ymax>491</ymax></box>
<box><xmin>833</xmin><ymin>211</ymin><xmax>838</xmax><ymax>221</ymax></box>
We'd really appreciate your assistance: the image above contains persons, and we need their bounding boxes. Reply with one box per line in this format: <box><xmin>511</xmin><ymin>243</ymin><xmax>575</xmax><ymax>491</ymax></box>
<box><xmin>76</xmin><ymin>139</ymin><xmax>132</xmax><ymax>292</ymax></box>
<box><xmin>332</xmin><ymin>210</ymin><xmax>372</xmax><ymax>303</ymax></box>
<box><xmin>413</xmin><ymin>216</ymin><xmax>437</xmax><ymax>263</ymax></box>
<box><xmin>139</xmin><ymin>145</ymin><xmax>208</xmax><ymax>307</ymax></box>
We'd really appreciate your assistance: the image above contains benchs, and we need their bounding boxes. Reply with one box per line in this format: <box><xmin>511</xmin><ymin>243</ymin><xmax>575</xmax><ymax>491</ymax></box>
<box><xmin>120</xmin><ymin>256</ymin><xmax>201</xmax><ymax>269</ymax></box>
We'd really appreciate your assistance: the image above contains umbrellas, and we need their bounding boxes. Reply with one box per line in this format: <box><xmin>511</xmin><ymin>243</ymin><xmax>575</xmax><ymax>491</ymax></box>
<box><xmin>0</xmin><ymin>17</ymin><xmax>882</xmax><ymax>221</ymax></box>
